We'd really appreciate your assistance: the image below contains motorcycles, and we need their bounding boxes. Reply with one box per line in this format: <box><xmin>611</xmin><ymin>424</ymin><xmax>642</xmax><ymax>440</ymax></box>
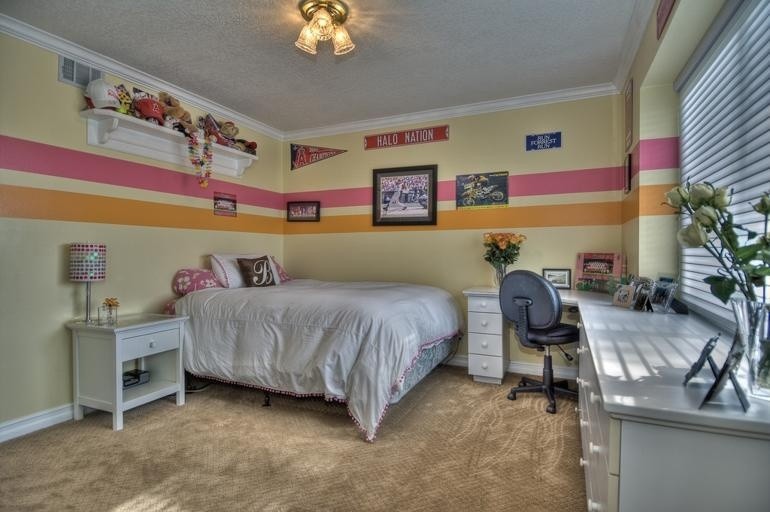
<box><xmin>458</xmin><ymin>178</ymin><xmax>505</xmax><ymax>207</ymax></box>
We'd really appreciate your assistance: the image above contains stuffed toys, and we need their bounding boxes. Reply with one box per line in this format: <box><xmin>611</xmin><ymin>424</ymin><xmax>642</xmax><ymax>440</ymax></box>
<box><xmin>85</xmin><ymin>79</ymin><xmax>257</xmax><ymax>154</ymax></box>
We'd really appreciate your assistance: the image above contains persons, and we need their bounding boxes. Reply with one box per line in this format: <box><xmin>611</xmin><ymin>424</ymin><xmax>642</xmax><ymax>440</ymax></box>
<box><xmin>290</xmin><ymin>205</ymin><xmax>316</xmax><ymax>217</ymax></box>
<box><xmin>468</xmin><ymin>175</ymin><xmax>488</xmax><ymax>191</ymax></box>
<box><xmin>381</xmin><ymin>175</ymin><xmax>429</xmax><ymax>218</ymax></box>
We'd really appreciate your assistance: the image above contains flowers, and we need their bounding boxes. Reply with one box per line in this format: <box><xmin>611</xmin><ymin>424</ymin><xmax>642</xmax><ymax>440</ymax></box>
<box><xmin>481</xmin><ymin>232</ymin><xmax>527</xmax><ymax>284</ymax></box>
<box><xmin>103</xmin><ymin>297</ymin><xmax>120</xmax><ymax>315</ymax></box>
<box><xmin>660</xmin><ymin>178</ymin><xmax>769</xmax><ymax>389</ymax></box>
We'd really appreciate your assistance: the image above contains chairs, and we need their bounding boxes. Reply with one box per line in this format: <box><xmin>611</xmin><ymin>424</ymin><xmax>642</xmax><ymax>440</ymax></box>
<box><xmin>499</xmin><ymin>268</ymin><xmax>579</xmax><ymax>413</ymax></box>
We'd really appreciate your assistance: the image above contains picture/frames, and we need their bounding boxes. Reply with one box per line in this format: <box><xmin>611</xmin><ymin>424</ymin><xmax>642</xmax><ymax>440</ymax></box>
<box><xmin>287</xmin><ymin>201</ymin><xmax>320</xmax><ymax>222</ymax></box>
<box><xmin>578</xmin><ymin>252</ymin><xmax>622</xmax><ymax>282</ymax></box>
<box><xmin>543</xmin><ymin>268</ymin><xmax>571</xmax><ymax>289</ymax></box>
<box><xmin>373</xmin><ymin>165</ymin><xmax>437</xmax><ymax>226</ymax></box>
<box><xmin>623</xmin><ymin>77</ymin><xmax>634</xmax><ymax>195</ymax></box>
<box><xmin>612</xmin><ymin>274</ymin><xmax>681</xmax><ymax>312</ymax></box>
<box><xmin>656</xmin><ymin>0</ymin><xmax>675</xmax><ymax>40</ymax></box>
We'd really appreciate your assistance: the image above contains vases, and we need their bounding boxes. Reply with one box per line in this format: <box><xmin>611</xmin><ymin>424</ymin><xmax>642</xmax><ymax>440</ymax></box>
<box><xmin>98</xmin><ymin>306</ymin><xmax>117</xmax><ymax>327</ymax></box>
<box><xmin>730</xmin><ymin>296</ymin><xmax>769</xmax><ymax>401</ymax></box>
<box><xmin>490</xmin><ymin>261</ymin><xmax>507</xmax><ymax>289</ymax></box>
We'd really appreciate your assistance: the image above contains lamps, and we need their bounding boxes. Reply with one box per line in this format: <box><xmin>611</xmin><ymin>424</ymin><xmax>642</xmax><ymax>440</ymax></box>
<box><xmin>294</xmin><ymin>0</ymin><xmax>356</xmax><ymax>55</ymax></box>
<box><xmin>68</xmin><ymin>243</ymin><xmax>107</xmax><ymax>322</ymax></box>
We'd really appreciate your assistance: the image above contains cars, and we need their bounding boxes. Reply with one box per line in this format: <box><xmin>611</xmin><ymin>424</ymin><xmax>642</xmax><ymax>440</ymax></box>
<box><xmin>418</xmin><ymin>195</ymin><xmax>428</xmax><ymax>209</ymax></box>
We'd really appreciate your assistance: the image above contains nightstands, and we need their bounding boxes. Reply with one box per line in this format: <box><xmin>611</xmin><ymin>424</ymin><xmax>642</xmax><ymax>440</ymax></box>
<box><xmin>65</xmin><ymin>312</ymin><xmax>191</xmax><ymax>431</ymax></box>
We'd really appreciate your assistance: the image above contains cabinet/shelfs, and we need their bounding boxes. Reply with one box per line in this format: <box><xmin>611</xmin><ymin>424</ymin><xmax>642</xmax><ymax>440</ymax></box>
<box><xmin>461</xmin><ymin>285</ymin><xmax>769</xmax><ymax>512</ymax></box>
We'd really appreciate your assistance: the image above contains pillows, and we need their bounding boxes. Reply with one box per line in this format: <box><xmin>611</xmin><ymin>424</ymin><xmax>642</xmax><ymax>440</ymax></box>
<box><xmin>206</xmin><ymin>253</ymin><xmax>263</xmax><ymax>288</ymax></box>
<box><xmin>173</xmin><ymin>266</ymin><xmax>224</xmax><ymax>295</ymax></box>
<box><xmin>236</xmin><ymin>254</ymin><xmax>281</xmax><ymax>286</ymax></box>
<box><xmin>270</xmin><ymin>256</ymin><xmax>291</xmax><ymax>282</ymax></box>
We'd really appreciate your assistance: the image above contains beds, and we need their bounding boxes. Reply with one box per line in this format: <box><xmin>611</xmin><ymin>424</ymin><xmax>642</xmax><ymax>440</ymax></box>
<box><xmin>165</xmin><ymin>277</ymin><xmax>466</xmax><ymax>443</ymax></box>
